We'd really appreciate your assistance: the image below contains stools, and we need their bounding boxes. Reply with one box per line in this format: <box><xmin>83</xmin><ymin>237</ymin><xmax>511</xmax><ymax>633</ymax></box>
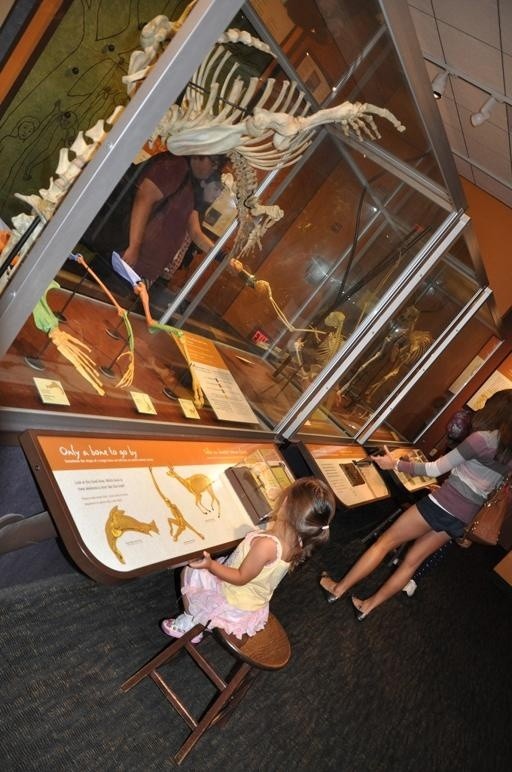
<box><xmin>122</xmin><ymin>612</ymin><xmax>290</xmax><ymax>767</ymax></box>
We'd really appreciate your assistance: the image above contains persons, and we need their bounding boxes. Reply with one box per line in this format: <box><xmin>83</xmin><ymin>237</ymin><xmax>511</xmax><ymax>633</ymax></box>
<box><xmin>160</xmin><ymin>473</ymin><xmax>336</xmax><ymax>646</ymax></box>
<box><xmin>316</xmin><ymin>391</ymin><xmax>512</xmax><ymax>621</ymax></box>
<box><xmin>146</xmin><ymin>170</ymin><xmax>245</xmax><ymax>305</ymax></box>
<box><xmin>97</xmin><ymin>139</ymin><xmax>225</xmax><ymax>308</ymax></box>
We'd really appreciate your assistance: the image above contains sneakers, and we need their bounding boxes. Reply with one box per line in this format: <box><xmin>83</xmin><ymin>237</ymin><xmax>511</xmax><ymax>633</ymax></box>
<box><xmin>162</xmin><ymin>618</ymin><xmax>203</xmax><ymax>643</ymax></box>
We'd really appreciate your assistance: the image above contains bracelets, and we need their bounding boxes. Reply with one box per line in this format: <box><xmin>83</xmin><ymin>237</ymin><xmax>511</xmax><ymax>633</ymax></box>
<box><xmin>393</xmin><ymin>460</ymin><xmax>399</xmax><ymax>474</ymax></box>
<box><xmin>208</xmin><ymin>244</ymin><xmax>227</xmax><ymax>261</ymax></box>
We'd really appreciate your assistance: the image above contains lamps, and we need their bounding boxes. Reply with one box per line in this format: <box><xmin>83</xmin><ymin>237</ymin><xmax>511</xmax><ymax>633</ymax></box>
<box><xmin>429</xmin><ymin>66</ymin><xmax>502</xmax><ymax>127</ymax></box>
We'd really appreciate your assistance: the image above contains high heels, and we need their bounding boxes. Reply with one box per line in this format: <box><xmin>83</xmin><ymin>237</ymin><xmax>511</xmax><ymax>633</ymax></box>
<box><xmin>317</xmin><ymin>567</ymin><xmax>340</xmax><ymax>605</ymax></box>
<box><xmin>350</xmin><ymin>591</ymin><xmax>368</xmax><ymax>622</ymax></box>
